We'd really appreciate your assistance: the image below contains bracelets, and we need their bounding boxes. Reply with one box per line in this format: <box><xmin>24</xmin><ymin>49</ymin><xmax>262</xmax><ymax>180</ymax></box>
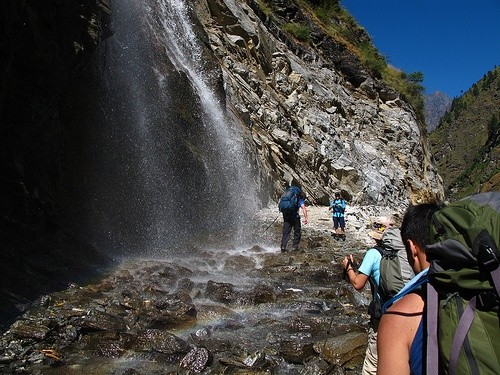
<box><xmin>346</xmin><ymin>268</ymin><xmax>356</xmax><ymax>273</ymax></box>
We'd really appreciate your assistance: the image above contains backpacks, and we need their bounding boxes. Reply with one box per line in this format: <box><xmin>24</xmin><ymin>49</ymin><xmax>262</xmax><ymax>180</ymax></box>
<box><xmin>378</xmin><ymin>228</ymin><xmax>416</xmax><ymax>302</ymax></box>
<box><xmin>423</xmin><ymin>190</ymin><xmax>500</xmax><ymax>375</ymax></box>
<box><xmin>278</xmin><ymin>191</ymin><xmax>299</xmax><ymax>210</ymax></box>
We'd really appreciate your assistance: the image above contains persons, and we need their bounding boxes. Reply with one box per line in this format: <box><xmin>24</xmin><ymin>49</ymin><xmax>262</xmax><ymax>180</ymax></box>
<box><xmin>342</xmin><ymin>216</ymin><xmax>393</xmax><ymax>375</ymax></box>
<box><xmin>277</xmin><ymin>174</ymin><xmax>308</xmax><ymax>252</ymax></box>
<box><xmin>329</xmin><ymin>191</ymin><xmax>346</xmax><ymax>241</ymax></box>
<box><xmin>375</xmin><ymin>201</ymin><xmax>447</xmax><ymax>375</ymax></box>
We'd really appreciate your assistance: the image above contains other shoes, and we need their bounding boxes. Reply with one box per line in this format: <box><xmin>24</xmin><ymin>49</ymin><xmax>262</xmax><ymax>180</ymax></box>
<box><xmin>292</xmin><ymin>245</ymin><xmax>299</xmax><ymax>251</ymax></box>
<box><xmin>280</xmin><ymin>245</ymin><xmax>287</xmax><ymax>252</ymax></box>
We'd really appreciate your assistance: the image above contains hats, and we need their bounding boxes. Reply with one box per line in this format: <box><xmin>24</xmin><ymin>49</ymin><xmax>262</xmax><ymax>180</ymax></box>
<box><xmin>335</xmin><ymin>192</ymin><xmax>340</xmax><ymax>196</ymax></box>
<box><xmin>369</xmin><ymin>217</ymin><xmax>393</xmax><ymax>240</ymax></box>
